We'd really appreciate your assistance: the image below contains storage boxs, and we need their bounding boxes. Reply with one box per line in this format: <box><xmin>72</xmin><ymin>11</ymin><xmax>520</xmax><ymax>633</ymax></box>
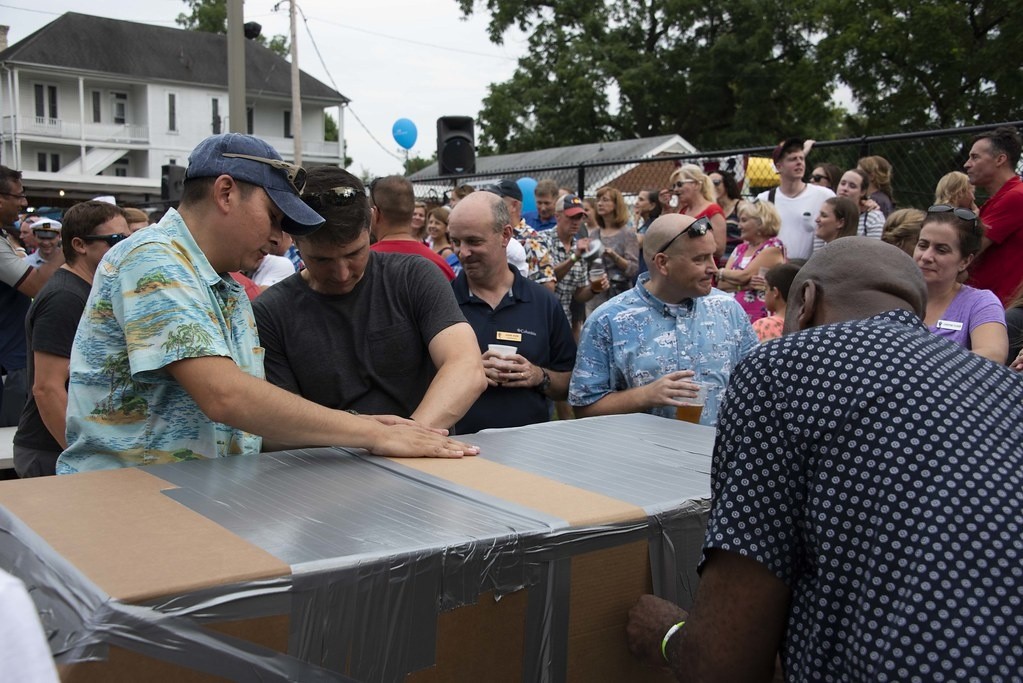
<box><xmin>0</xmin><ymin>412</ymin><xmax>787</xmax><ymax>683</ymax></box>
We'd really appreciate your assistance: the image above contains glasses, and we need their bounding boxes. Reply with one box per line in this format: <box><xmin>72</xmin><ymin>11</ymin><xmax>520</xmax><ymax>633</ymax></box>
<box><xmin>223</xmin><ymin>152</ymin><xmax>308</xmax><ymax>195</ymax></box>
<box><xmin>927</xmin><ymin>204</ymin><xmax>979</xmax><ymax>248</ymax></box>
<box><xmin>652</xmin><ymin>216</ymin><xmax>713</xmax><ymax>261</ymax></box>
<box><xmin>78</xmin><ymin>233</ymin><xmax>127</xmax><ymax>248</ymax></box>
<box><xmin>0</xmin><ymin>192</ymin><xmax>27</xmax><ymax>200</ymax></box>
<box><xmin>25</xmin><ymin>218</ymin><xmax>35</xmax><ymax>224</ymax></box>
<box><xmin>673</xmin><ymin>178</ymin><xmax>697</xmax><ymax>190</ymax></box>
<box><xmin>712</xmin><ymin>179</ymin><xmax>725</xmax><ymax>185</ymax></box>
<box><xmin>300</xmin><ymin>186</ymin><xmax>366</xmax><ymax>213</ymax></box>
<box><xmin>369</xmin><ymin>175</ymin><xmax>384</xmax><ymax>213</ymax></box>
<box><xmin>808</xmin><ymin>175</ymin><xmax>831</xmax><ymax>182</ymax></box>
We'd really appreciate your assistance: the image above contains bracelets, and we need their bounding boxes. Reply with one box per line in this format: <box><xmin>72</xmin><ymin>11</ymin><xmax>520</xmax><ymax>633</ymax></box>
<box><xmin>718</xmin><ymin>267</ymin><xmax>724</xmax><ymax>281</ymax></box>
<box><xmin>541</xmin><ymin>368</ymin><xmax>551</xmax><ymax>391</ymax></box>
<box><xmin>591</xmin><ymin>288</ymin><xmax>600</xmax><ymax>294</ymax></box>
<box><xmin>571</xmin><ymin>254</ymin><xmax>577</xmax><ymax>262</ymax></box>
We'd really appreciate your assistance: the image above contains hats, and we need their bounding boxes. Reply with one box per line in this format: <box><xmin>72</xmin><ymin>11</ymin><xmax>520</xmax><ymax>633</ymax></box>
<box><xmin>774</xmin><ymin>138</ymin><xmax>804</xmax><ymax>164</ymax></box>
<box><xmin>185</xmin><ymin>132</ymin><xmax>325</xmax><ymax>236</ymax></box>
<box><xmin>488</xmin><ymin>179</ymin><xmax>523</xmax><ymax>201</ymax></box>
<box><xmin>29</xmin><ymin>219</ymin><xmax>63</xmax><ymax>238</ymax></box>
<box><xmin>555</xmin><ymin>194</ymin><xmax>588</xmax><ymax>219</ymax></box>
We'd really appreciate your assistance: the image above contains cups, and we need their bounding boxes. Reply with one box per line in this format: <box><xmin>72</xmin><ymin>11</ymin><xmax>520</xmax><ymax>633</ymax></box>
<box><xmin>488</xmin><ymin>343</ymin><xmax>518</xmax><ymax>383</ymax></box>
<box><xmin>754</xmin><ymin>267</ymin><xmax>770</xmax><ymax>290</ymax></box>
<box><xmin>590</xmin><ymin>269</ymin><xmax>604</xmax><ymax>292</ymax></box>
<box><xmin>677</xmin><ymin>382</ymin><xmax>708</xmax><ymax>424</ymax></box>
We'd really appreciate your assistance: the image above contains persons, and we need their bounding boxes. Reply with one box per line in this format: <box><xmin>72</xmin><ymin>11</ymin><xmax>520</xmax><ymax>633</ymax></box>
<box><xmin>625</xmin><ymin>235</ymin><xmax>1023</xmax><ymax>683</ymax></box>
<box><xmin>0</xmin><ymin>130</ymin><xmax>1023</xmax><ymax>476</ymax></box>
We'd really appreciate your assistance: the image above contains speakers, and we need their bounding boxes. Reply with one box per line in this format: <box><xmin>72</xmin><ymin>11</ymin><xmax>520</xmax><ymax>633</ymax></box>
<box><xmin>436</xmin><ymin>116</ymin><xmax>475</xmax><ymax>175</ymax></box>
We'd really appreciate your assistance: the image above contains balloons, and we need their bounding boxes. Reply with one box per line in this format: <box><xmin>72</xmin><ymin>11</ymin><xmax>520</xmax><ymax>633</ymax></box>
<box><xmin>514</xmin><ymin>177</ymin><xmax>538</xmax><ymax>214</ymax></box>
<box><xmin>392</xmin><ymin>119</ymin><xmax>418</xmax><ymax>150</ymax></box>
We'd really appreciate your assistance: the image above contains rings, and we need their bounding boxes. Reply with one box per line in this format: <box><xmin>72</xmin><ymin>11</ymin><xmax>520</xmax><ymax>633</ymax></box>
<box><xmin>521</xmin><ymin>373</ymin><xmax>524</xmax><ymax>379</ymax></box>
